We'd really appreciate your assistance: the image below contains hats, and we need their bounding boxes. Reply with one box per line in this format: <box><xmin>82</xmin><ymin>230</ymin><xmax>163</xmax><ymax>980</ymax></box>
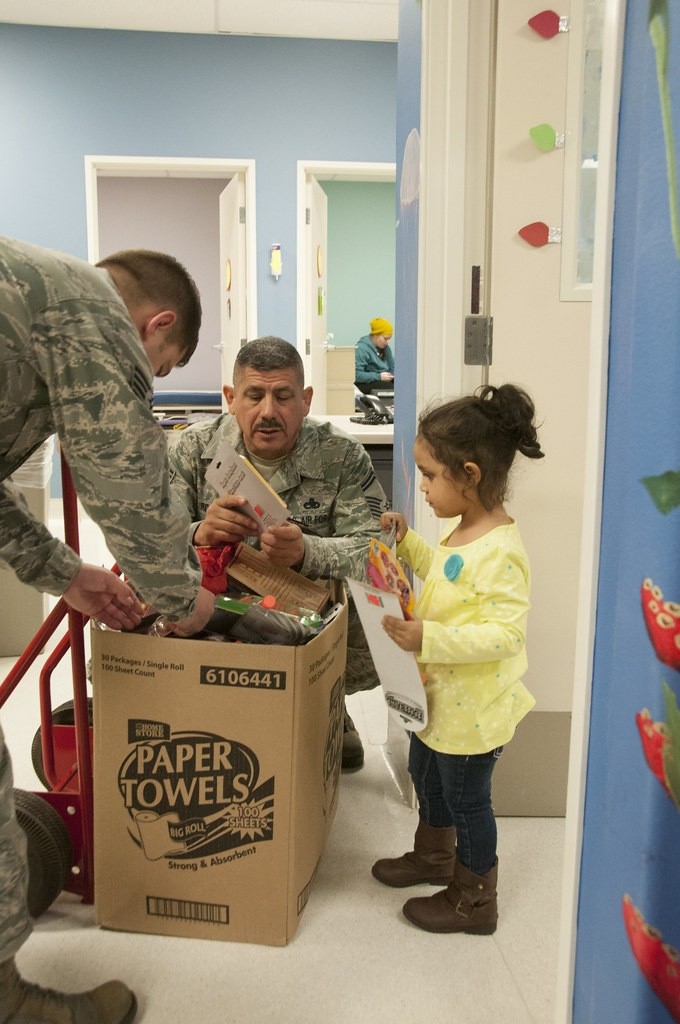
<box><xmin>369</xmin><ymin>317</ymin><xmax>393</xmax><ymax>336</ymax></box>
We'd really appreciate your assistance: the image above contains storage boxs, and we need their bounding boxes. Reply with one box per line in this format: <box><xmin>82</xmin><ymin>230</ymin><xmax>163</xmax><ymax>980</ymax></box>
<box><xmin>89</xmin><ymin>577</ymin><xmax>352</xmax><ymax>949</ymax></box>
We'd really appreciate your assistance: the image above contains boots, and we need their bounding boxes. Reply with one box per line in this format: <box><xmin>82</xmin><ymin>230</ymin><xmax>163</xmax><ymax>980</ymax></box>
<box><xmin>403</xmin><ymin>854</ymin><xmax>499</xmax><ymax>936</ymax></box>
<box><xmin>342</xmin><ymin>707</ymin><xmax>364</xmax><ymax>768</ymax></box>
<box><xmin>0</xmin><ymin>956</ymin><xmax>138</xmax><ymax>1024</ymax></box>
<box><xmin>371</xmin><ymin>819</ymin><xmax>457</xmax><ymax>886</ymax></box>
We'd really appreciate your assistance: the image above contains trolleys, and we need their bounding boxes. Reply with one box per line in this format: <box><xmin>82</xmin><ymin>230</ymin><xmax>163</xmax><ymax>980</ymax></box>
<box><xmin>0</xmin><ymin>436</ymin><xmax>129</xmax><ymax>907</ymax></box>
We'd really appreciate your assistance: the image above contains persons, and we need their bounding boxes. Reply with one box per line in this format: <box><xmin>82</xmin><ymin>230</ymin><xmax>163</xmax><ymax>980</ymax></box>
<box><xmin>371</xmin><ymin>380</ymin><xmax>551</xmax><ymax>936</ymax></box>
<box><xmin>1</xmin><ymin>224</ymin><xmax>219</xmax><ymax>1024</ymax></box>
<box><xmin>157</xmin><ymin>336</ymin><xmax>397</xmax><ymax>772</ymax></box>
<box><xmin>355</xmin><ymin>317</ymin><xmax>396</xmax><ymax>395</ymax></box>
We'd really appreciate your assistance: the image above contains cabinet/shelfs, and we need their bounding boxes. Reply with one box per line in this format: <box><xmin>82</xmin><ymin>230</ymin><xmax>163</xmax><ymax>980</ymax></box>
<box><xmin>326</xmin><ymin>344</ymin><xmax>359</xmax><ymax>417</ymax></box>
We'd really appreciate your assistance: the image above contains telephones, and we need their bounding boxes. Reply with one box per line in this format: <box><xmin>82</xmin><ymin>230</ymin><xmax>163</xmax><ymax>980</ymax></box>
<box><xmin>355</xmin><ymin>395</ymin><xmax>394</xmax><ymax>424</ymax></box>
